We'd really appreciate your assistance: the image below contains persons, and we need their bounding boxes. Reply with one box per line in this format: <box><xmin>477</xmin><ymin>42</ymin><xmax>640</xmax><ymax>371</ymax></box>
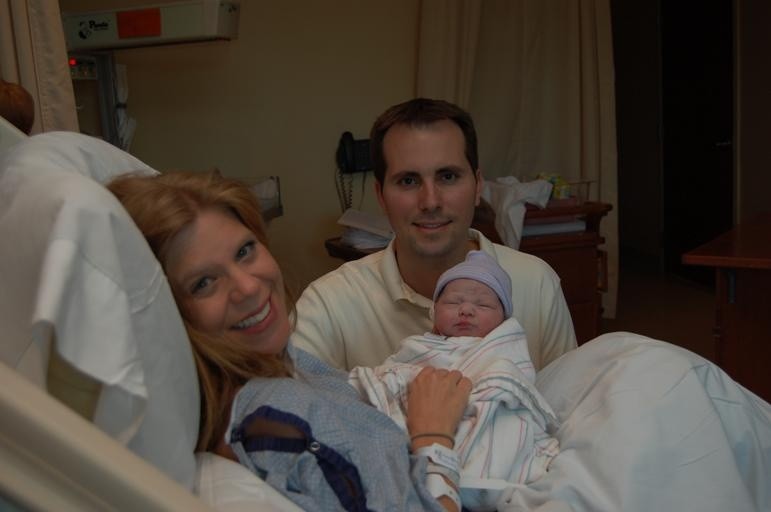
<box><xmin>104</xmin><ymin>166</ymin><xmax>771</xmax><ymax>510</ymax></box>
<box><xmin>355</xmin><ymin>247</ymin><xmax>557</xmax><ymax>487</ymax></box>
<box><xmin>285</xmin><ymin>100</ymin><xmax>578</xmax><ymax>388</ymax></box>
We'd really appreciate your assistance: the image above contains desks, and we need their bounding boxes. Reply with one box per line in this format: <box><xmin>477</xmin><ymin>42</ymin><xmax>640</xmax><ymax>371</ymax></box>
<box><xmin>681</xmin><ymin>212</ymin><xmax>771</xmax><ymax>365</ymax></box>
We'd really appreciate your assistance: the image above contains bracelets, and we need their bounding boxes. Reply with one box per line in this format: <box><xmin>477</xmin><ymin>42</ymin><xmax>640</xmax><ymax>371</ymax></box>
<box><xmin>404</xmin><ymin>433</ymin><xmax>459</xmax><ymax>442</ymax></box>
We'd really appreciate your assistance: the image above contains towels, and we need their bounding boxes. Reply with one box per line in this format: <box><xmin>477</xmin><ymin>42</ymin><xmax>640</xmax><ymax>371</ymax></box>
<box><xmin>480</xmin><ymin>176</ymin><xmax>552</xmax><ymax>251</ymax></box>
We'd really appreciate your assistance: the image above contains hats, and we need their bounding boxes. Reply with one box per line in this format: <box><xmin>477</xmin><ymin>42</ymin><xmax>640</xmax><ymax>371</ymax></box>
<box><xmin>432</xmin><ymin>249</ymin><xmax>513</xmax><ymax>319</ymax></box>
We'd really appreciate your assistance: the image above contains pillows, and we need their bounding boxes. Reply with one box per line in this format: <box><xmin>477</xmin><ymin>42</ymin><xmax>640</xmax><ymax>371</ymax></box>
<box><xmin>0</xmin><ymin>130</ymin><xmax>203</xmax><ymax>500</ymax></box>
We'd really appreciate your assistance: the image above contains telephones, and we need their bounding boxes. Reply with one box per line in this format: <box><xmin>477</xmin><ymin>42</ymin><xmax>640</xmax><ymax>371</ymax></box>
<box><xmin>335</xmin><ymin>132</ymin><xmax>373</xmax><ymax>174</ymax></box>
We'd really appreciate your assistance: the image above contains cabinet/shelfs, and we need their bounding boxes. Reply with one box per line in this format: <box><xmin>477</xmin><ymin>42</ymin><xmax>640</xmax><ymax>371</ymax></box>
<box><xmin>473</xmin><ymin>202</ymin><xmax>613</xmax><ymax>347</ymax></box>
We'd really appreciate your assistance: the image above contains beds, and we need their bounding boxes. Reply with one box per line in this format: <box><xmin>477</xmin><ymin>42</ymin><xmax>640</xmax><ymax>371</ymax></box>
<box><xmin>0</xmin><ymin>113</ymin><xmax>308</xmax><ymax>512</ymax></box>
